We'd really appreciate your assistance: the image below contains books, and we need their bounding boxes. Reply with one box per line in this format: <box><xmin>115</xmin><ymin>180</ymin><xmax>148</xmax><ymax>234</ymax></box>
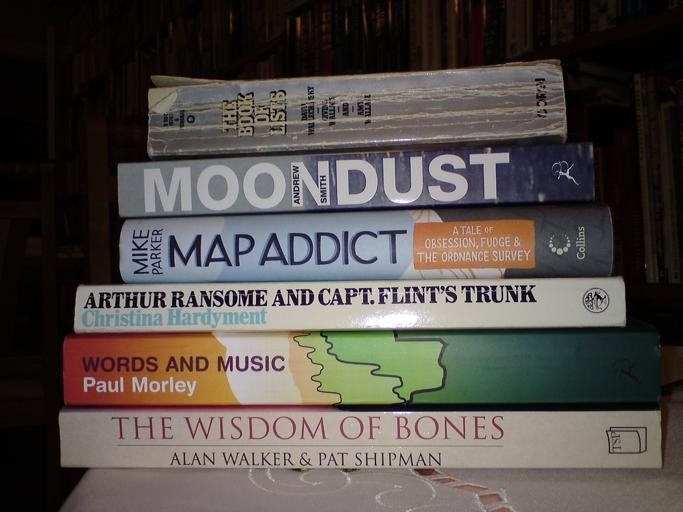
<box><xmin>2</xmin><ymin>3</ymin><xmax>679</xmax><ymax>470</ymax></box>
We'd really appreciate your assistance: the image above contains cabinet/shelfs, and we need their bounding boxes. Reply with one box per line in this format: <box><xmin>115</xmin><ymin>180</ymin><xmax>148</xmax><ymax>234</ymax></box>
<box><xmin>70</xmin><ymin>1</ymin><xmax>682</xmax><ymax>403</ymax></box>
<box><xmin>1</xmin><ymin>23</ymin><xmax>60</xmax><ymax>509</ymax></box>
